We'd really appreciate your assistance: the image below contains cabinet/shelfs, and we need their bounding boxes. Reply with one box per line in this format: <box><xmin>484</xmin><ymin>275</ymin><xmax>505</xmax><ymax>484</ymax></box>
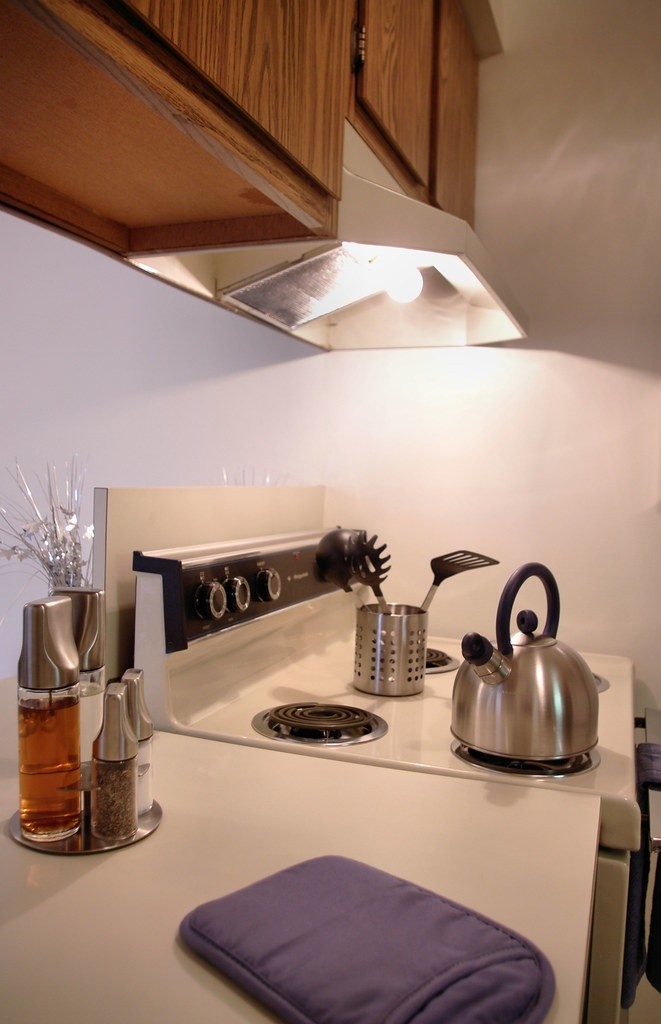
<box><xmin>0</xmin><ymin>0</ymin><xmax>363</xmax><ymax>263</ymax></box>
<box><xmin>344</xmin><ymin>0</ymin><xmax>502</xmax><ymax>227</ymax></box>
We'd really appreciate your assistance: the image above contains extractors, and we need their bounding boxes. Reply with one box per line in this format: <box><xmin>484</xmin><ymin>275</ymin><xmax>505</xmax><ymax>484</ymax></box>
<box><xmin>217</xmin><ymin>119</ymin><xmax>531</xmax><ymax>350</ymax></box>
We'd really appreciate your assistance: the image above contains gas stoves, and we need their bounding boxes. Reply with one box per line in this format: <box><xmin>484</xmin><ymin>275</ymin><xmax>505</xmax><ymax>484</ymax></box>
<box><xmin>132</xmin><ymin>529</ymin><xmax>642</xmax><ymax>850</ymax></box>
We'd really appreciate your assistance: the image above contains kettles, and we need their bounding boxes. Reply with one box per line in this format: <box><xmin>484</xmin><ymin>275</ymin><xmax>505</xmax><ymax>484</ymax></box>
<box><xmin>447</xmin><ymin>560</ymin><xmax>601</xmax><ymax>759</ymax></box>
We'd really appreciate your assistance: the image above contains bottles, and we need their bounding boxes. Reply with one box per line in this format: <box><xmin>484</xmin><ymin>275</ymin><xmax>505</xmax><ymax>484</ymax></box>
<box><xmin>90</xmin><ymin>683</ymin><xmax>138</xmax><ymax>839</ymax></box>
<box><xmin>49</xmin><ymin>587</ymin><xmax>107</xmax><ymax>790</ymax></box>
<box><xmin>122</xmin><ymin>668</ymin><xmax>154</xmax><ymax>814</ymax></box>
<box><xmin>17</xmin><ymin>597</ymin><xmax>81</xmax><ymax>841</ymax></box>
